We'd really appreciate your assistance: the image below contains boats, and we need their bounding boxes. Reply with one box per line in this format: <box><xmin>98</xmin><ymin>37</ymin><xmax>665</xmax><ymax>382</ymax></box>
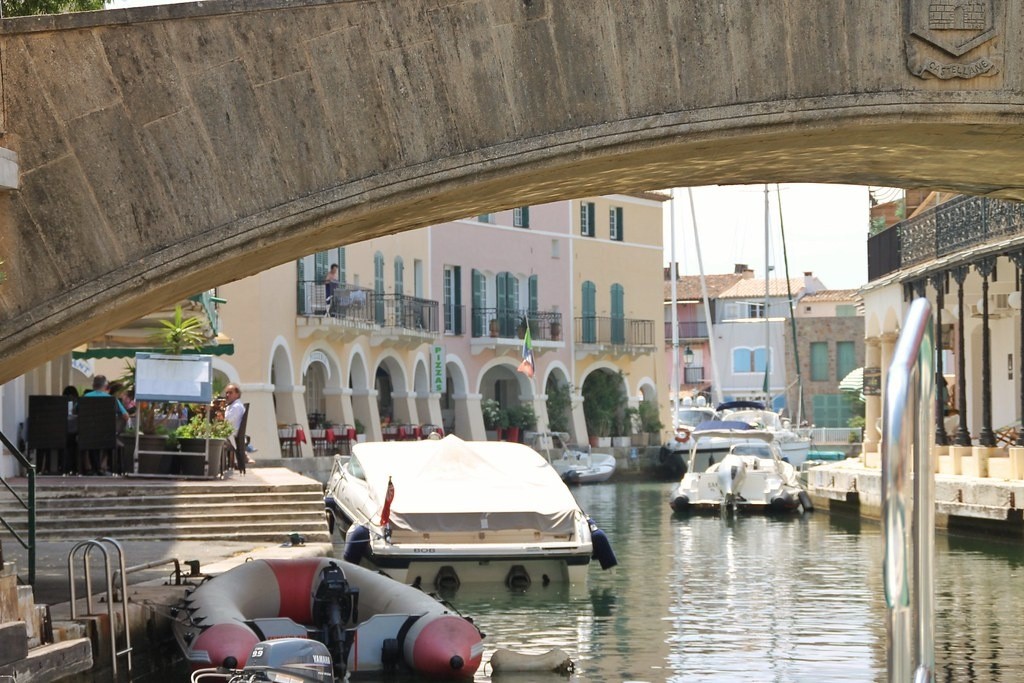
<box><xmin>169</xmin><ymin>556</ymin><xmax>487</xmax><ymax>683</ymax></box>
<box><xmin>658</xmin><ymin>378</ymin><xmax>811</xmax><ymax>482</ymax></box>
<box><xmin>527</xmin><ymin>432</ymin><xmax>617</xmax><ymax>484</ymax></box>
<box><xmin>669</xmin><ymin>429</ymin><xmax>802</xmax><ymax>513</ymax></box>
<box><xmin>324</xmin><ymin>432</ymin><xmax>618</xmax><ymax>587</ymax></box>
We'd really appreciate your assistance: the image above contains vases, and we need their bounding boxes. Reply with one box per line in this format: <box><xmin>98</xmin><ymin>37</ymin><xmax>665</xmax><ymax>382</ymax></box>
<box><xmin>516</xmin><ymin>324</ymin><xmax>527</xmax><ymax>339</ymax></box>
<box><xmin>490</xmin><ymin>319</ymin><xmax>501</xmax><ymax>338</ymax></box>
<box><xmin>551</xmin><ymin>323</ymin><xmax>561</xmax><ymax>341</ymax></box>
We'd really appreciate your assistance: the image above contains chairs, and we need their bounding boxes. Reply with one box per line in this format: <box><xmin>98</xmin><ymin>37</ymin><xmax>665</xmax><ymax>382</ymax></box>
<box><xmin>28</xmin><ymin>395</ymin><xmax>77</xmax><ymax>476</ymax></box>
<box><xmin>72</xmin><ymin>396</ymin><xmax>120</xmax><ymax>475</ymax></box>
<box><xmin>220</xmin><ymin>402</ymin><xmax>250</xmax><ymax>479</ymax></box>
<box><xmin>308</xmin><ymin>284</ymin><xmax>335</xmax><ymax>318</ymax></box>
<box><xmin>277</xmin><ymin>424</ymin><xmax>444</xmax><ymax>458</ymax></box>
<box><xmin>946</xmin><ymin>420</ymin><xmax>1022</xmax><ymax>451</ymax></box>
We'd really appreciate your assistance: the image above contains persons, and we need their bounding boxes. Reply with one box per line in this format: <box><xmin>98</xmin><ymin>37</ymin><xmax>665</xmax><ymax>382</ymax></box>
<box><xmin>326</xmin><ymin>263</ymin><xmax>338</xmax><ymax>279</ymax></box>
<box><xmin>934</xmin><ymin>373</ymin><xmax>948</xmax><ymax>415</ymax></box>
<box><xmin>63</xmin><ymin>375</ymin><xmax>257</xmax><ymax>476</ymax></box>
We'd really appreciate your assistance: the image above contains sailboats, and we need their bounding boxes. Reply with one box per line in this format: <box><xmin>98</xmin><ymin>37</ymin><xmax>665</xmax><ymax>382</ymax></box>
<box><xmin>662</xmin><ymin>187</ymin><xmax>725</xmax><ymax>425</ymax></box>
<box><xmin>749</xmin><ymin>183</ymin><xmax>846</xmax><ymax>465</ymax></box>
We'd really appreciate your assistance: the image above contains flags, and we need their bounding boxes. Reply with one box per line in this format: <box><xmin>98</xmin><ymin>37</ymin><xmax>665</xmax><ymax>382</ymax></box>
<box><xmin>381</xmin><ymin>481</ymin><xmax>395</xmax><ymax>526</ymax></box>
<box><xmin>518</xmin><ymin>321</ymin><xmax>534</xmax><ymax>378</ymax></box>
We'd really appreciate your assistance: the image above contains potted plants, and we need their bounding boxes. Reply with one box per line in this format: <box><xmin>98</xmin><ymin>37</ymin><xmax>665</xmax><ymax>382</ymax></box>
<box><xmin>504</xmin><ymin>402</ymin><xmax>522</xmax><ymax>443</ymax></box>
<box><xmin>491</xmin><ymin>409</ymin><xmax>509</xmax><ymax>440</ymax></box>
<box><xmin>113</xmin><ymin>301</ymin><xmax>210</xmax><ymax>476</ymax></box>
<box><xmin>485</xmin><ymin>430</ymin><xmax>499</xmax><ymax>442</ymax></box>
<box><xmin>170</xmin><ymin>414</ymin><xmax>234</xmax><ymax>481</ymax></box>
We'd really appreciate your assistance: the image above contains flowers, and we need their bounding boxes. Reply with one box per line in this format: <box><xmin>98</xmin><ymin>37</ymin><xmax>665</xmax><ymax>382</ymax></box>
<box><xmin>480</xmin><ymin>398</ymin><xmax>498</xmax><ymax>429</ymax></box>
<box><xmin>519</xmin><ymin>401</ymin><xmax>540</xmax><ymax>426</ymax></box>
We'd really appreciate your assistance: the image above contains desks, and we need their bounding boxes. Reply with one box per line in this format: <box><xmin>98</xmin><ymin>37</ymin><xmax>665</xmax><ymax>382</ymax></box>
<box><xmin>277</xmin><ymin>425</ymin><xmax>302</xmax><ymax>457</ymax></box>
<box><xmin>332</xmin><ymin>426</ymin><xmax>353</xmax><ymax>455</ymax></box>
<box><xmin>309</xmin><ymin>429</ymin><xmax>326</xmax><ymax>457</ymax></box>
<box><xmin>399</xmin><ymin>426</ymin><xmax>418</xmax><ymax>441</ymax></box>
<box><xmin>381</xmin><ymin>425</ymin><xmax>398</xmax><ymax>441</ymax></box>
<box><xmin>421</xmin><ymin>425</ymin><xmax>442</xmax><ymax>439</ymax></box>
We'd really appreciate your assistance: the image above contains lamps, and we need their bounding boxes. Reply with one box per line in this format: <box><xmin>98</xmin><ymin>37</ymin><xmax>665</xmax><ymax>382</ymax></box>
<box><xmin>933</xmin><ymin>290</ymin><xmax>1021</xmax><ymax>323</ymax></box>
